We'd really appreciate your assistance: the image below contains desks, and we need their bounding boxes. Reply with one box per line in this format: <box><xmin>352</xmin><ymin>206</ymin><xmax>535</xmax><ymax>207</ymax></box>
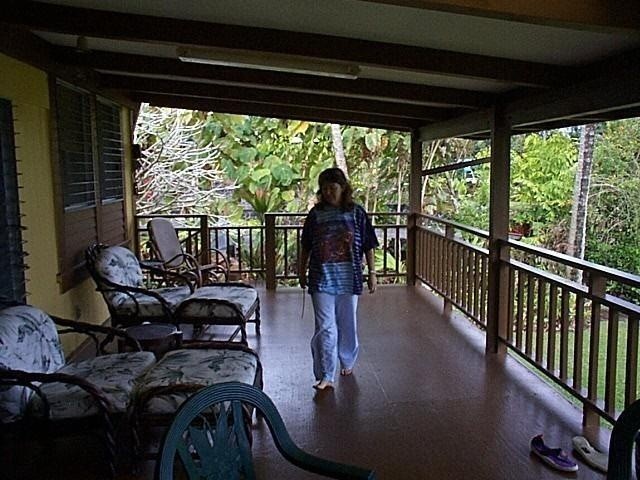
<box><xmin>116</xmin><ymin>326</ymin><xmax>182</xmax><ymax>353</ymax></box>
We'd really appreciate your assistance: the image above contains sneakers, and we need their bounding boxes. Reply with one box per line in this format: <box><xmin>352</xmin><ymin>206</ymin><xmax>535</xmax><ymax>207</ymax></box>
<box><xmin>572</xmin><ymin>436</ymin><xmax>608</xmax><ymax>472</ymax></box>
<box><xmin>531</xmin><ymin>435</ymin><xmax>579</xmax><ymax>471</ymax></box>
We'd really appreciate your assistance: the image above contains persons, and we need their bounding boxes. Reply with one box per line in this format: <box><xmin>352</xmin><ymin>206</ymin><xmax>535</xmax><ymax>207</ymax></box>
<box><xmin>299</xmin><ymin>167</ymin><xmax>379</xmax><ymax>390</ymax></box>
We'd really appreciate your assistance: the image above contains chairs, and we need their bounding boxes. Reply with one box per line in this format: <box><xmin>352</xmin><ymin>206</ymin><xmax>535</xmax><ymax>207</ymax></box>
<box><xmin>1</xmin><ymin>299</ymin><xmax>155</xmax><ymax>479</ymax></box>
<box><xmin>141</xmin><ymin>218</ymin><xmax>231</xmax><ymax>287</ymax></box>
<box><xmin>154</xmin><ymin>382</ymin><xmax>376</xmax><ymax>480</ymax></box>
<box><xmin>86</xmin><ymin>241</ymin><xmax>195</xmax><ymax>344</ymax></box>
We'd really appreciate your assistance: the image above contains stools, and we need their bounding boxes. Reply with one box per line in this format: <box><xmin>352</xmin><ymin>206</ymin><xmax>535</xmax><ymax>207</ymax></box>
<box><xmin>175</xmin><ymin>283</ymin><xmax>261</xmax><ymax>350</ymax></box>
<box><xmin>132</xmin><ymin>343</ymin><xmax>262</xmax><ymax>480</ymax></box>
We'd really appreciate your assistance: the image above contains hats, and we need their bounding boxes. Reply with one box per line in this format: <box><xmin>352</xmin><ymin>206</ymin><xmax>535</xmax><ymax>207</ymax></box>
<box><xmin>319</xmin><ymin>168</ymin><xmax>346</xmax><ymax>185</ymax></box>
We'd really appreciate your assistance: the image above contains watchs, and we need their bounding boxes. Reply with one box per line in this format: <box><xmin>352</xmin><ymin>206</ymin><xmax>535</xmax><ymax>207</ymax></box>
<box><xmin>368</xmin><ymin>270</ymin><xmax>376</xmax><ymax>274</ymax></box>
